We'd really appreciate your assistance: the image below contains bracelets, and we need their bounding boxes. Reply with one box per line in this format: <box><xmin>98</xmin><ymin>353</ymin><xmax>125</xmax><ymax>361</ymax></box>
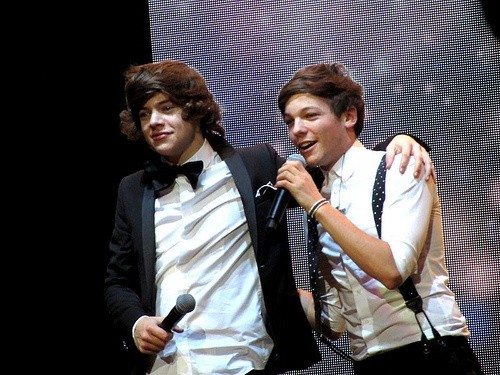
<box><xmin>308</xmin><ymin>198</ymin><xmax>329</xmax><ymax>218</ymax></box>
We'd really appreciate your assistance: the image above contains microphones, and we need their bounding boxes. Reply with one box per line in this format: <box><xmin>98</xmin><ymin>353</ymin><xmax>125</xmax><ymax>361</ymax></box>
<box><xmin>158</xmin><ymin>294</ymin><xmax>197</xmax><ymax>333</ymax></box>
<box><xmin>264</xmin><ymin>154</ymin><xmax>307</xmax><ymax>233</ymax></box>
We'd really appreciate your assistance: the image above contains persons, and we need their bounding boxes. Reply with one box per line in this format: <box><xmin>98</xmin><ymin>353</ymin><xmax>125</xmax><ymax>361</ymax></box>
<box><xmin>275</xmin><ymin>63</ymin><xmax>484</xmax><ymax>375</ymax></box>
<box><xmin>105</xmin><ymin>61</ymin><xmax>436</xmax><ymax>375</ymax></box>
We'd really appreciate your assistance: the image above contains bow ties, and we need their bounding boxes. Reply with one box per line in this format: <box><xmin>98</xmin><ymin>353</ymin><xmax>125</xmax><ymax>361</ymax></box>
<box><xmin>153</xmin><ymin>160</ymin><xmax>205</xmax><ymax>194</ymax></box>
